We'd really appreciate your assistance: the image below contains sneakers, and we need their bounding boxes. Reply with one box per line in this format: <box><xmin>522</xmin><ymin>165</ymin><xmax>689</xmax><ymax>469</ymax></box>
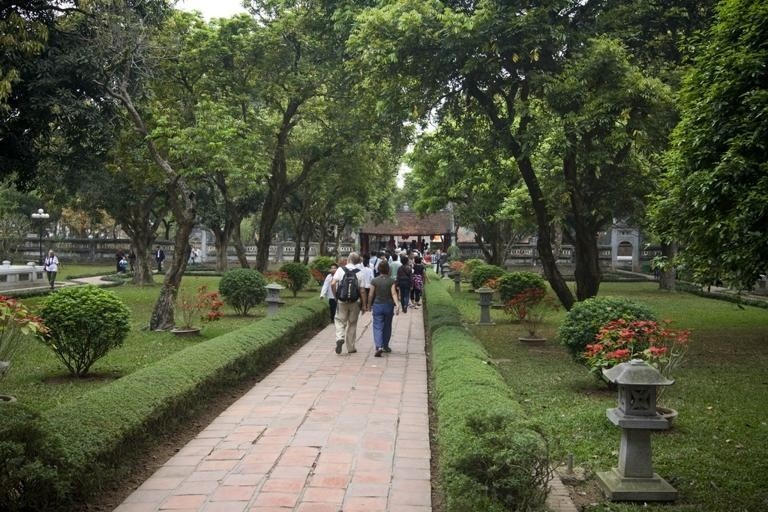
<box><xmin>348</xmin><ymin>348</ymin><xmax>358</xmax><ymax>353</ymax></box>
<box><xmin>334</xmin><ymin>338</ymin><xmax>344</xmax><ymax>355</ymax></box>
<box><xmin>373</xmin><ymin>347</ymin><xmax>382</xmax><ymax>358</ymax></box>
<box><xmin>382</xmin><ymin>345</ymin><xmax>393</xmax><ymax>352</ymax></box>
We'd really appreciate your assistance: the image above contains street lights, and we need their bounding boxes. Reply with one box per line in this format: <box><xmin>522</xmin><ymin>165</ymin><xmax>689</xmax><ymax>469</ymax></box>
<box><xmin>32</xmin><ymin>208</ymin><xmax>52</xmax><ymax>268</ymax></box>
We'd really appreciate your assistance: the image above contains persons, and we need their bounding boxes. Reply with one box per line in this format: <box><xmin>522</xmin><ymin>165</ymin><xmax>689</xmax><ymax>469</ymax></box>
<box><xmin>119</xmin><ymin>256</ymin><xmax>128</xmax><ymax>273</ymax></box>
<box><xmin>45</xmin><ymin>249</ymin><xmax>60</xmax><ymax>289</ymax></box>
<box><xmin>155</xmin><ymin>246</ymin><xmax>165</xmax><ymax>271</ymax></box>
<box><xmin>190</xmin><ymin>245</ymin><xmax>197</xmax><ymax>264</ymax></box>
<box><xmin>319</xmin><ymin>237</ymin><xmax>448</xmax><ymax>357</ymax></box>
<box><xmin>115</xmin><ymin>248</ymin><xmax>127</xmax><ymax>273</ymax></box>
<box><xmin>126</xmin><ymin>247</ymin><xmax>136</xmax><ymax>272</ymax></box>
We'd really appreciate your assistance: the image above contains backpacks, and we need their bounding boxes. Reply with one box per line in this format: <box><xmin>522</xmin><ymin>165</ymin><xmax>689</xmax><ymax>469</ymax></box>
<box><xmin>336</xmin><ymin>266</ymin><xmax>363</xmax><ymax>304</ymax></box>
